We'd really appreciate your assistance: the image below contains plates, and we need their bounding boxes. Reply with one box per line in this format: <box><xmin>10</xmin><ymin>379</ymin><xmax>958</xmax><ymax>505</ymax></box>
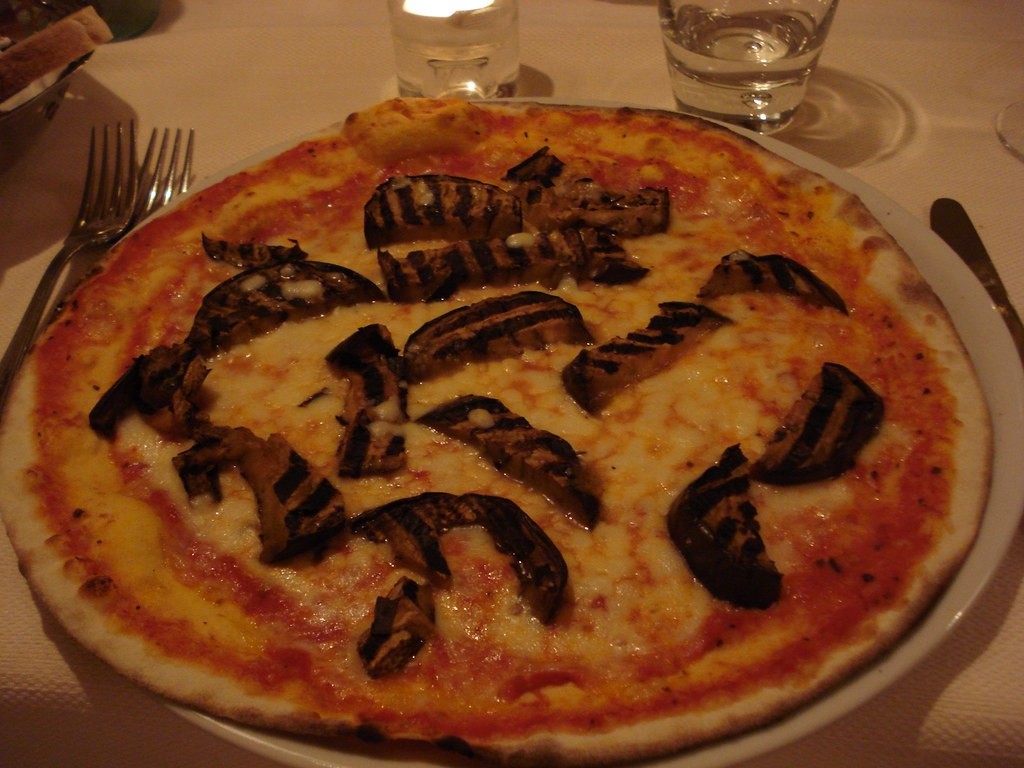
<box><xmin>129</xmin><ymin>99</ymin><xmax>1024</xmax><ymax>768</ymax></box>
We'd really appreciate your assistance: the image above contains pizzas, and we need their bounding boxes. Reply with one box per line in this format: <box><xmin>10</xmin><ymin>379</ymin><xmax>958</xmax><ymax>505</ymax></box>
<box><xmin>1</xmin><ymin>100</ymin><xmax>992</xmax><ymax>766</ymax></box>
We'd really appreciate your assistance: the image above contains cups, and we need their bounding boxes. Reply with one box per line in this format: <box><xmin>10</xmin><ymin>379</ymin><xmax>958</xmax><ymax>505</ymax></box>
<box><xmin>661</xmin><ymin>1</ymin><xmax>838</xmax><ymax>135</ymax></box>
<box><xmin>386</xmin><ymin>0</ymin><xmax>521</xmax><ymax>107</ymax></box>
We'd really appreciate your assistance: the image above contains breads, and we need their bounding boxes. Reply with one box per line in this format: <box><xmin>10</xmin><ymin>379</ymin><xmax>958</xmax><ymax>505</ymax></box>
<box><xmin>0</xmin><ymin>6</ymin><xmax>113</xmax><ymax>94</ymax></box>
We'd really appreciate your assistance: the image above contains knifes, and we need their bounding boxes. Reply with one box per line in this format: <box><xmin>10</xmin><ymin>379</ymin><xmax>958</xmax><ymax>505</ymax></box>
<box><xmin>931</xmin><ymin>198</ymin><xmax>1023</xmax><ymax>367</ymax></box>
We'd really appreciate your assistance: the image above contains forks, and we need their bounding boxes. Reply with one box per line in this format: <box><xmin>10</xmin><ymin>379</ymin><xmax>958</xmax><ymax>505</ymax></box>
<box><xmin>0</xmin><ymin>119</ymin><xmax>136</xmax><ymax>409</ymax></box>
<box><xmin>131</xmin><ymin>127</ymin><xmax>195</xmax><ymax>231</ymax></box>
